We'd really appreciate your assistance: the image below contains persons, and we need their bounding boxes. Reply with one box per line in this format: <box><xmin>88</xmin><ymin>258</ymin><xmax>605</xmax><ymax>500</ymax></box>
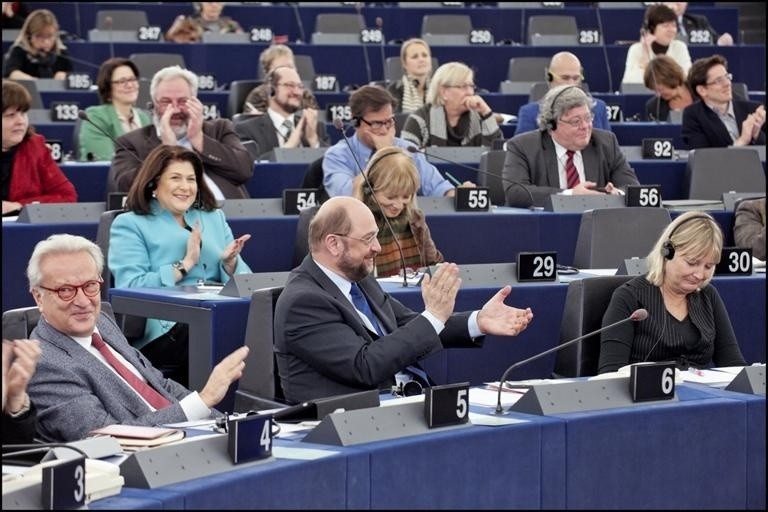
<box><xmin>22</xmin><ymin>232</ymin><xmax>252</xmax><ymax>440</ymax></box>
<box><xmin>351</xmin><ymin>146</ymin><xmax>443</xmax><ymax>280</ymax></box>
<box><xmin>274</xmin><ymin>192</ymin><xmax>535</xmax><ymax>407</ymax></box>
<box><xmin>2</xmin><ymin>334</ymin><xmax>54</xmax><ymax>461</ymax></box>
<box><xmin>104</xmin><ymin>143</ymin><xmax>256</xmax><ymax>392</ymax></box>
<box><xmin>733</xmin><ymin>197</ymin><xmax>767</xmax><ymax>265</ymax></box>
<box><xmin>2</xmin><ymin>2</ymin><xmax>768</xmax><ymax>219</ymax></box>
<box><xmin>599</xmin><ymin>210</ymin><xmax>749</xmax><ymax>376</ymax></box>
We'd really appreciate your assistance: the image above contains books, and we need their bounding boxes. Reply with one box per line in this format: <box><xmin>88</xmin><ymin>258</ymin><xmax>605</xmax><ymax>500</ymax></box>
<box><xmin>89</xmin><ymin>422</ymin><xmax>177</xmax><ymax>442</ymax></box>
<box><xmin>86</xmin><ymin>428</ymin><xmax>187</xmax><ymax>452</ymax></box>
<box><xmin>482</xmin><ymin>376</ymin><xmax>537</xmax><ymax>394</ymax></box>
<box><xmin>682</xmin><ymin>365</ymin><xmax>751</xmax><ymax>389</ymax></box>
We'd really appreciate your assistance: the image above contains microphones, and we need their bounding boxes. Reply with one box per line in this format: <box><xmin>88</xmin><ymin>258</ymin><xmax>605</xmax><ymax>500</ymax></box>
<box><xmin>640</xmin><ymin>28</ymin><xmax>663</xmax><ymax>124</ymax></box>
<box><xmin>407</xmin><ymin>146</ymin><xmax>544</xmax><ymax>210</ymax></box>
<box><xmin>375</xmin><ymin>17</ymin><xmax>388</xmax><ymax>87</ymax></box>
<box><xmin>39</xmin><ymin>47</ymin><xmax>100</xmax><ymax>68</ymax></box>
<box><xmin>105</xmin><ymin>15</ymin><xmax>115</xmax><ymax>57</ymax></box>
<box><xmin>333</xmin><ymin>118</ymin><xmax>415</xmax><ymax>288</ymax></box>
<box><xmin>79</xmin><ymin>111</ymin><xmax>144</xmax><ymax>162</ymax></box>
<box><xmin>489</xmin><ymin>308</ymin><xmax>648</xmax><ymax>416</ymax></box>
<box><xmin>594</xmin><ymin>2</ymin><xmax>613</xmax><ymax>93</ymax></box>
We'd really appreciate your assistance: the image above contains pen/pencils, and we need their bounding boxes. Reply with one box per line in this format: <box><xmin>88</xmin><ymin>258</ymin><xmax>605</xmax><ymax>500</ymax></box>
<box><xmin>446</xmin><ymin>172</ymin><xmax>462</xmax><ymax>187</ymax></box>
<box><xmin>688</xmin><ymin>367</ymin><xmax>704</xmax><ymax>376</ymax></box>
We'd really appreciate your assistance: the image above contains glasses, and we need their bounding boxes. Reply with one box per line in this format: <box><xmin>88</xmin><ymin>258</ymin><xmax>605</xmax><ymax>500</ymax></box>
<box><xmin>274</xmin><ymin>81</ymin><xmax>305</xmax><ymax>91</ymax></box>
<box><xmin>39</xmin><ymin>276</ymin><xmax>105</xmax><ymax>302</ymax></box>
<box><xmin>558</xmin><ymin>111</ymin><xmax>596</xmax><ymax>128</ymax></box>
<box><xmin>361</xmin><ymin>115</ymin><xmax>395</xmax><ymax>129</ymax></box>
<box><xmin>706</xmin><ymin>72</ymin><xmax>733</xmax><ymax>87</ymax></box>
<box><xmin>111</xmin><ymin>75</ymin><xmax>138</xmax><ymax>87</ymax></box>
<box><xmin>335</xmin><ymin>227</ymin><xmax>379</xmax><ymax>246</ymax></box>
<box><xmin>441</xmin><ymin>82</ymin><xmax>478</xmax><ymax>91</ymax></box>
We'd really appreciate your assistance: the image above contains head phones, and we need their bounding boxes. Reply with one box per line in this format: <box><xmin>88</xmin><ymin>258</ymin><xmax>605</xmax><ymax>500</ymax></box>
<box><xmin>544</xmin><ymin>66</ymin><xmax>553</xmax><ymax>82</ymax></box>
<box><xmin>351</xmin><ymin>116</ymin><xmax>361</xmax><ymax>127</ymax></box>
<box><xmin>546</xmin><ymin>86</ymin><xmax>589</xmax><ymax>131</ymax></box>
<box><xmin>379</xmin><ymin>381</ymin><xmax>423</xmax><ymax>397</ymax></box>
<box><xmin>661</xmin><ymin>215</ymin><xmax>721</xmax><ymax>260</ymax></box>
<box><xmin>267</xmin><ymin>65</ymin><xmax>294</xmax><ymax>96</ymax></box>
<box><xmin>645</xmin><ymin>4</ymin><xmax>654</xmax><ymax>34</ymax></box>
<box><xmin>216</xmin><ymin>411</ymin><xmax>280</xmax><ymax>436</ymax></box>
<box><xmin>403</xmin><ymin>69</ymin><xmax>419</xmax><ymax>87</ymax></box>
<box><xmin>195</xmin><ymin>2</ymin><xmax>203</xmax><ymax>12</ymax></box>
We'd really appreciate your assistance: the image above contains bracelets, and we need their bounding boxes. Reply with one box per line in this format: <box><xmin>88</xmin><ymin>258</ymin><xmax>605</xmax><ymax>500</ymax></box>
<box><xmin>173</xmin><ymin>259</ymin><xmax>189</xmax><ymax>277</ymax></box>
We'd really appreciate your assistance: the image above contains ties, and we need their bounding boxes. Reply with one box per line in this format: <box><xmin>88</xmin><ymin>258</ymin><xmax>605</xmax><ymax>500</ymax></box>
<box><xmin>565</xmin><ymin>149</ymin><xmax>581</xmax><ymax>190</ymax></box>
<box><xmin>92</xmin><ymin>332</ymin><xmax>173</xmax><ymax>410</ymax></box>
<box><xmin>351</xmin><ymin>281</ymin><xmax>431</xmax><ymax>389</ymax></box>
<box><xmin>283</xmin><ymin>119</ymin><xmax>296</xmax><ymax>138</ymax></box>
